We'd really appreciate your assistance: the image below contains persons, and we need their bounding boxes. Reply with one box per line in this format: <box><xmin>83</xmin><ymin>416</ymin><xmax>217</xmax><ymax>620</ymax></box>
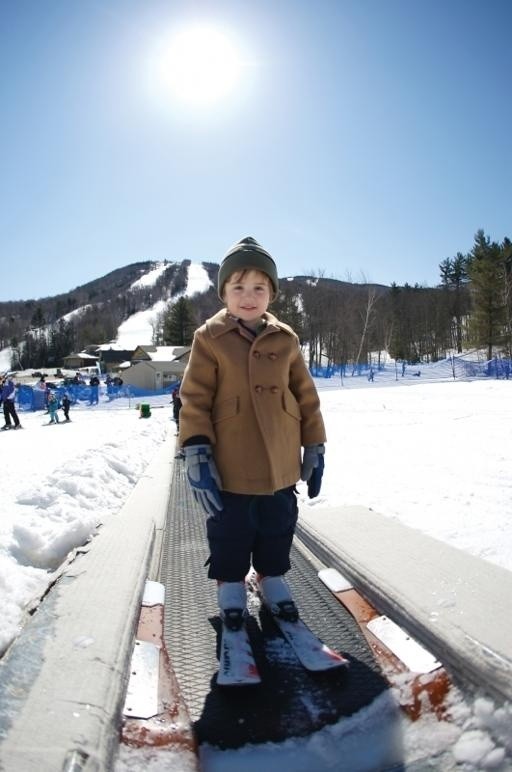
<box><xmin>0</xmin><ymin>367</ymin><xmax>181</xmax><ymax>431</ymax></box>
<box><xmin>367</xmin><ymin>368</ymin><xmax>375</xmax><ymax>382</ymax></box>
<box><xmin>401</xmin><ymin>362</ymin><xmax>407</xmax><ymax>376</ymax></box>
<box><xmin>177</xmin><ymin>233</ymin><xmax>328</xmax><ymax>630</ymax></box>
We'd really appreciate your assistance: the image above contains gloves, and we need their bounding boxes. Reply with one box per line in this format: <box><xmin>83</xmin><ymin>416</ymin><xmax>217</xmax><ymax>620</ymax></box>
<box><xmin>179</xmin><ymin>444</ymin><xmax>224</xmax><ymax>518</ymax></box>
<box><xmin>301</xmin><ymin>444</ymin><xmax>325</xmax><ymax>498</ymax></box>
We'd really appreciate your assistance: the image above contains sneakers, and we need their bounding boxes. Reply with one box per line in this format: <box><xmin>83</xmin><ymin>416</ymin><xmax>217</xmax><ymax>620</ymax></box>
<box><xmin>256</xmin><ymin>572</ymin><xmax>299</xmax><ymax>609</ymax></box>
<box><xmin>217</xmin><ymin>582</ymin><xmax>247</xmax><ymax>611</ymax></box>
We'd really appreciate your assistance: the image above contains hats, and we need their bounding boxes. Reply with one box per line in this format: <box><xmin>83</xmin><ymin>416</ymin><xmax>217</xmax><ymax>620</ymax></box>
<box><xmin>218</xmin><ymin>237</ymin><xmax>280</xmax><ymax>304</ymax></box>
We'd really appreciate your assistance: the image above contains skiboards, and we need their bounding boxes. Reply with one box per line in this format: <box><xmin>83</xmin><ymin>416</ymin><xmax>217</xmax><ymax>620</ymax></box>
<box><xmin>217</xmin><ymin>566</ymin><xmax>349</xmax><ymax>685</ymax></box>
